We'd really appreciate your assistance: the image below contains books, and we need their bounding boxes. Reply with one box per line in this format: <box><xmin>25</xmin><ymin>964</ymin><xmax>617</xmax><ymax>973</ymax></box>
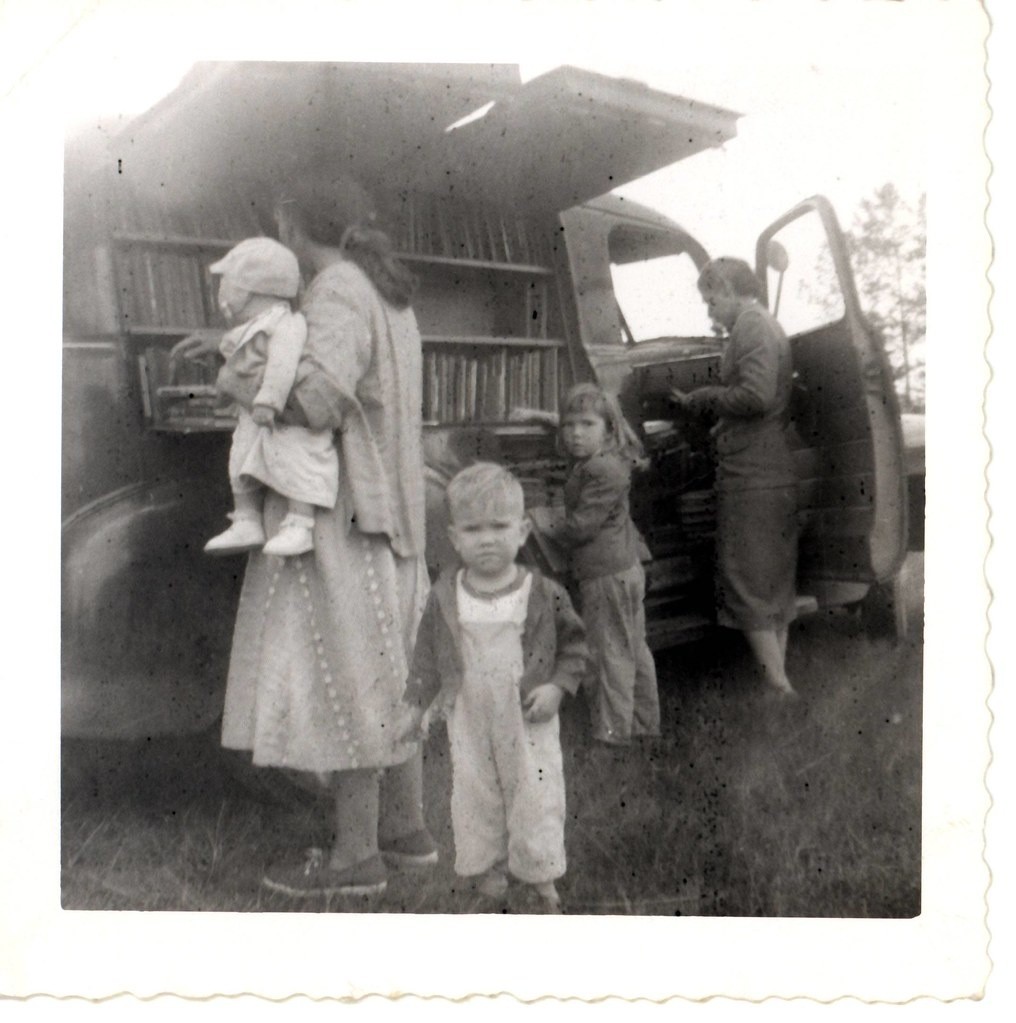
<box><xmin>113</xmin><ymin>247</ymin><xmax>248</xmax><ymax>330</ymax></box>
<box><xmin>120</xmin><ymin>178</ymin><xmax>553</xmax><ymax>269</ymax></box>
<box><xmin>134</xmin><ymin>343</ymin><xmax>566</xmax><ymax>431</ymax></box>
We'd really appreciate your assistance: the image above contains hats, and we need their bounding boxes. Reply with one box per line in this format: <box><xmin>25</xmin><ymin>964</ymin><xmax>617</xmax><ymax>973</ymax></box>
<box><xmin>209</xmin><ymin>237</ymin><xmax>300</xmax><ymax>298</ymax></box>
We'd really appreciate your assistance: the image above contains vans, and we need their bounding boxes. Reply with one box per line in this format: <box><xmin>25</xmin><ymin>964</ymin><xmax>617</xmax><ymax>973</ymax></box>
<box><xmin>61</xmin><ymin>55</ymin><xmax>909</xmax><ymax>753</ymax></box>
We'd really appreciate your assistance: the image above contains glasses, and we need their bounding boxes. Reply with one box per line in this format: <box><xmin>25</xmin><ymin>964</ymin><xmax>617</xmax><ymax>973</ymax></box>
<box><xmin>272</xmin><ymin>198</ymin><xmax>299</xmax><ymax>222</ymax></box>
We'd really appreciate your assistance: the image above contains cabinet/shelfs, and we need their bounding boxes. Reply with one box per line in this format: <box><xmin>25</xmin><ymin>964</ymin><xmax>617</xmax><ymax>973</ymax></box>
<box><xmin>115</xmin><ymin>229</ymin><xmax>567</xmax><ymax>426</ymax></box>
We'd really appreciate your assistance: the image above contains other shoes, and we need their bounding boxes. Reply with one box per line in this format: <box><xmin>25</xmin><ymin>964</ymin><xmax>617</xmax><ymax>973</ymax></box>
<box><xmin>458</xmin><ymin>876</ymin><xmax>510</xmax><ymax>915</ymax></box>
<box><xmin>747</xmin><ymin>690</ymin><xmax>808</xmax><ymax>721</ymax></box>
<box><xmin>629</xmin><ymin>732</ymin><xmax>671</xmax><ymax>758</ymax></box>
<box><xmin>377</xmin><ymin>826</ymin><xmax>439</xmax><ymax>866</ymax></box>
<box><xmin>260</xmin><ymin>852</ymin><xmax>389</xmax><ymax>897</ymax></box>
<box><xmin>203</xmin><ymin>519</ymin><xmax>266</xmax><ymax>555</ymax></box>
<box><xmin>262</xmin><ymin>515</ymin><xmax>314</xmax><ymax>558</ymax></box>
<box><xmin>572</xmin><ymin>738</ymin><xmax>632</xmax><ymax>764</ymax></box>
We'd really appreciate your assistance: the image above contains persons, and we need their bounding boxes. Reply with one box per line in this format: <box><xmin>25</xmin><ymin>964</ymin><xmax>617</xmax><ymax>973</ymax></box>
<box><xmin>166</xmin><ymin>165</ymin><xmax>438</xmax><ymax>896</ymax></box>
<box><xmin>680</xmin><ymin>257</ymin><xmax>802</xmax><ymax>696</ymax></box>
<box><xmin>506</xmin><ymin>383</ymin><xmax>661</xmax><ymax>747</ymax></box>
<box><xmin>388</xmin><ymin>461</ymin><xmax>588</xmax><ymax>915</ymax></box>
